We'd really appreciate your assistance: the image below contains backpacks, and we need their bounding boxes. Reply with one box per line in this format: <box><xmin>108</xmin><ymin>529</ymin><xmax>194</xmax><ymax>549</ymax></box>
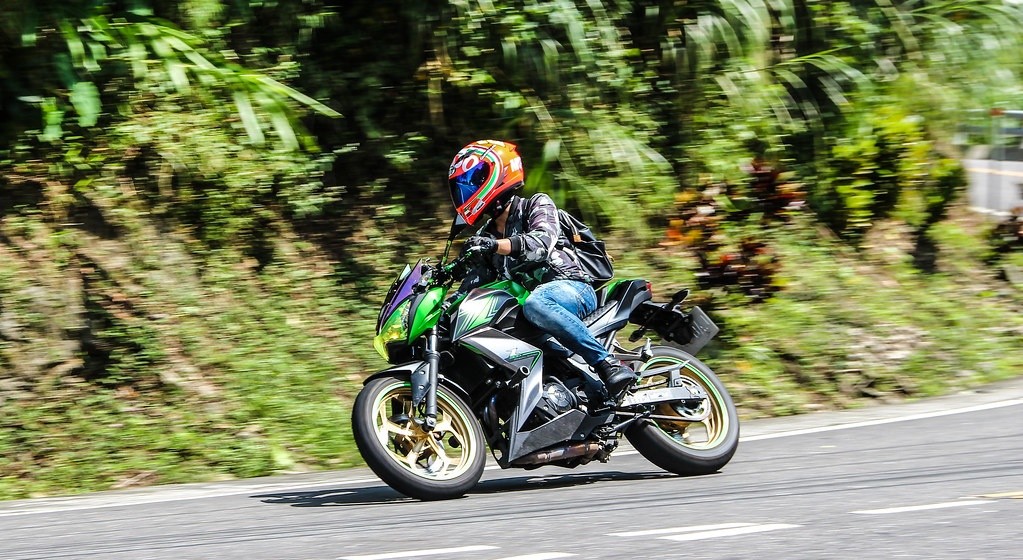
<box><xmin>522</xmin><ymin>193</ymin><xmax>614</xmax><ymax>290</ymax></box>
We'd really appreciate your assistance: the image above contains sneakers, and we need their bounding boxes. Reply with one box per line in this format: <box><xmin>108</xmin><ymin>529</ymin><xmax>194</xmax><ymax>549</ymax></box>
<box><xmin>594</xmin><ymin>354</ymin><xmax>638</xmax><ymax>395</ymax></box>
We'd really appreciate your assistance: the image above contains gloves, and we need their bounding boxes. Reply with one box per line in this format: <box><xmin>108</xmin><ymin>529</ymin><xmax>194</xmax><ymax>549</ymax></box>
<box><xmin>421</xmin><ymin>265</ymin><xmax>451</xmax><ymax>282</ymax></box>
<box><xmin>459</xmin><ymin>236</ymin><xmax>498</xmax><ymax>268</ymax></box>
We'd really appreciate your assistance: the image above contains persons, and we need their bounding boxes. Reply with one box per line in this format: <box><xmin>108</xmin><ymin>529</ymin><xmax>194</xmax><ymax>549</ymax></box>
<box><xmin>449</xmin><ymin>139</ymin><xmax>638</xmax><ymax>395</ymax></box>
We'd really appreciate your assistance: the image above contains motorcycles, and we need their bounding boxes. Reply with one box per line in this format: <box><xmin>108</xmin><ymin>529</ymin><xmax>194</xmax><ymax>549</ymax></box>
<box><xmin>351</xmin><ymin>212</ymin><xmax>739</xmax><ymax>501</ymax></box>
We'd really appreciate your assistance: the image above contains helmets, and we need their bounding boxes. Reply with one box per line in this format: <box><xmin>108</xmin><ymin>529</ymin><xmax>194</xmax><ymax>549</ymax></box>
<box><xmin>448</xmin><ymin>140</ymin><xmax>524</xmax><ymax>225</ymax></box>
